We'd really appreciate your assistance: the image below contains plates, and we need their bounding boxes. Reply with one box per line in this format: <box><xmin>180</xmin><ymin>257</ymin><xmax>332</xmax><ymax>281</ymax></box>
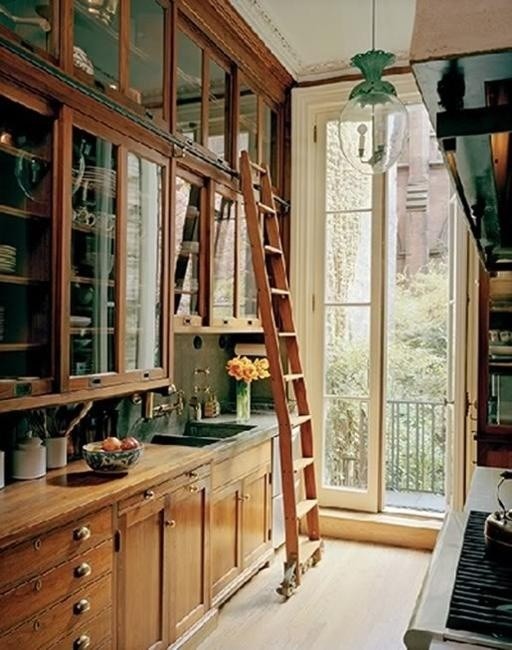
<box><xmin>125</xmin><ymin>151</ymin><xmax>141</xmax><ymax>370</ymax></box>
<box><xmin>0</xmin><ymin>245</ymin><xmax>17</xmax><ymax>273</ymax></box>
<box><xmin>489</xmin><ymin>345</ymin><xmax>512</xmax><ymax>355</ymax></box>
<box><xmin>73</xmin><ymin>165</ymin><xmax>117</xmax><ymax>192</ymax></box>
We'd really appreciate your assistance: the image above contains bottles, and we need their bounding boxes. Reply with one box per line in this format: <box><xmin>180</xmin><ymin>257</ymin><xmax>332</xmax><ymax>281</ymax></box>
<box><xmin>188</xmin><ymin>394</ymin><xmax>203</xmax><ymax>422</ymax></box>
<box><xmin>10</xmin><ymin>429</ymin><xmax>47</xmax><ymax>481</ymax></box>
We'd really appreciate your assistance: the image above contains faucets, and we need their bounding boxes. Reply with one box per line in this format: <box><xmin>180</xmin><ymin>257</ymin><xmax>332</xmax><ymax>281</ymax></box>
<box><xmin>160</xmin><ymin>389</ymin><xmax>184</xmax><ymax>416</ymax></box>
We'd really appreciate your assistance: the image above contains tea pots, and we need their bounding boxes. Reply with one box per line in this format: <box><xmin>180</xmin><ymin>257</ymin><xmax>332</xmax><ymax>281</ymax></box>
<box><xmin>484</xmin><ymin>470</ymin><xmax>512</xmax><ymax>548</ymax></box>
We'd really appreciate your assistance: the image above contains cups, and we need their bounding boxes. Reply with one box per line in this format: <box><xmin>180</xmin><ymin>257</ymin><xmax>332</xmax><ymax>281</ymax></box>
<box><xmin>43</xmin><ymin>436</ymin><xmax>68</xmax><ymax>470</ymax></box>
<box><xmin>488</xmin><ymin>329</ymin><xmax>512</xmax><ymax>343</ymax></box>
<box><xmin>94</xmin><ymin>211</ymin><xmax>116</xmax><ymax>231</ymax></box>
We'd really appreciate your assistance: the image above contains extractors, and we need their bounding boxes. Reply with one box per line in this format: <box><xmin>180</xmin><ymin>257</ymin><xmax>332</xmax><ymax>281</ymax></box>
<box><xmin>407</xmin><ymin>0</ymin><xmax>512</xmax><ymax>273</ymax></box>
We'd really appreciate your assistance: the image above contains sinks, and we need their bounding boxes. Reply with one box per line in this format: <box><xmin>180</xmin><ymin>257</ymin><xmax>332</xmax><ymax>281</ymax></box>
<box><xmin>139</xmin><ymin>434</ymin><xmax>220</xmax><ymax>449</ymax></box>
<box><xmin>160</xmin><ymin>423</ymin><xmax>258</xmax><ymax>440</ymax></box>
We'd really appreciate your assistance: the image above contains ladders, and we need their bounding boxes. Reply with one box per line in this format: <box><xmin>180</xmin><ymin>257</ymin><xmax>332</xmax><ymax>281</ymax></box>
<box><xmin>240</xmin><ymin>150</ymin><xmax>324</xmax><ymax>598</ymax></box>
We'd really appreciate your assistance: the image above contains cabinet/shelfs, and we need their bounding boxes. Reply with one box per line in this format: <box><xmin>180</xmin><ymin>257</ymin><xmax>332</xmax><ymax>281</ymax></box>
<box><xmin>471</xmin><ymin>259</ymin><xmax>512</xmax><ymax>468</ymax></box>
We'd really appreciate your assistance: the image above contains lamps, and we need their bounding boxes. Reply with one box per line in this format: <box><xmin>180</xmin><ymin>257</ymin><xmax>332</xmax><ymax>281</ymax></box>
<box><xmin>338</xmin><ymin>1</ymin><xmax>409</xmax><ymax>175</ymax></box>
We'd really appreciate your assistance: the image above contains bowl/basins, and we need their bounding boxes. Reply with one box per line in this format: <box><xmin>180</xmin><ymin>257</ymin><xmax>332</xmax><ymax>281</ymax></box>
<box><xmin>69</xmin><ymin>315</ymin><xmax>92</xmax><ymax>327</ymax></box>
<box><xmin>185</xmin><ymin>205</ymin><xmax>199</xmax><ymax>219</ymax></box>
<box><xmin>81</xmin><ymin>442</ymin><xmax>144</xmax><ymax>475</ymax></box>
<box><xmin>182</xmin><ymin>241</ymin><xmax>199</xmax><ymax>255</ymax></box>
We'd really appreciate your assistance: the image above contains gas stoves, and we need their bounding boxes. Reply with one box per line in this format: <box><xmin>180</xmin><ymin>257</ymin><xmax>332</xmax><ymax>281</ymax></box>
<box><xmin>446</xmin><ymin>509</ymin><xmax>512</xmax><ymax>637</ymax></box>
